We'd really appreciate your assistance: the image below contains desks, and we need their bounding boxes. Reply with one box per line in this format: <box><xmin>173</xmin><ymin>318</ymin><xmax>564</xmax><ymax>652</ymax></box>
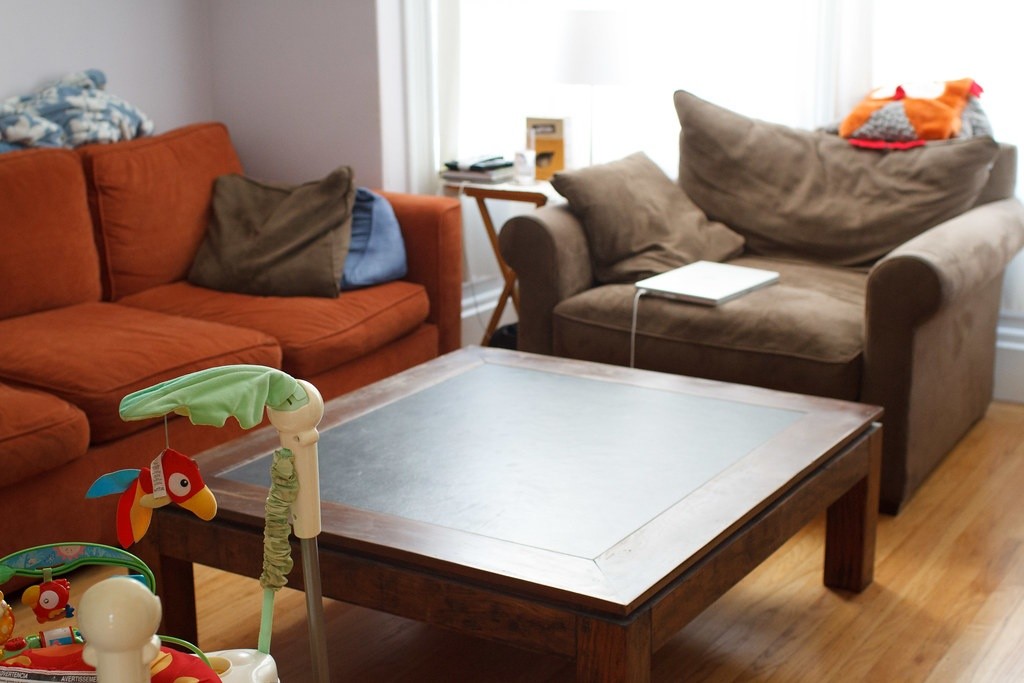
<box><xmin>436</xmin><ymin>168</ymin><xmax>566</xmax><ymax>345</ymax></box>
<box><xmin>137</xmin><ymin>342</ymin><xmax>881</xmax><ymax>683</ymax></box>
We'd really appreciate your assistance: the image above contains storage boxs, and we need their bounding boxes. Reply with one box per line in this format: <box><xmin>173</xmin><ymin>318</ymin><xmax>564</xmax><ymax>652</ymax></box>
<box><xmin>528</xmin><ymin>116</ymin><xmax>564</xmax><ymax>184</ymax></box>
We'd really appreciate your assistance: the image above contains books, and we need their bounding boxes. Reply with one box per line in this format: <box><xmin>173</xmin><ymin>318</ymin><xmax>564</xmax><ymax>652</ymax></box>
<box><xmin>442</xmin><ymin>157</ymin><xmax>516</xmax><ymax>183</ymax></box>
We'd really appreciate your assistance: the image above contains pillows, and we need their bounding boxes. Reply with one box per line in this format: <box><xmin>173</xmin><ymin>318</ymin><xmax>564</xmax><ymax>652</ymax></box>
<box><xmin>671</xmin><ymin>89</ymin><xmax>1001</xmax><ymax>266</ymax></box>
<box><xmin>343</xmin><ymin>189</ymin><xmax>407</xmax><ymax>289</ymax></box>
<box><xmin>549</xmin><ymin>153</ymin><xmax>748</xmax><ymax>283</ymax></box>
<box><xmin>185</xmin><ymin>167</ymin><xmax>356</xmax><ymax>298</ymax></box>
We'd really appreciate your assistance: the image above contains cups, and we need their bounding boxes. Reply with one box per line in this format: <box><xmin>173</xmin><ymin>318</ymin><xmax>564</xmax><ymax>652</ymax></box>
<box><xmin>514</xmin><ymin>128</ymin><xmax>536</xmax><ymax>184</ymax></box>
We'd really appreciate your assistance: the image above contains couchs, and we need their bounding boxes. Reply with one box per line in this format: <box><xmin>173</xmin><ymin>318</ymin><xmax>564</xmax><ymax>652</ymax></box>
<box><xmin>501</xmin><ymin>93</ymin><xmax>1023</xmax><ymax>518</ymax></box>
<box><xmin>0</xmin><ymin>122</ymin><xmax>463</xmax><ymax>593</ymax></box>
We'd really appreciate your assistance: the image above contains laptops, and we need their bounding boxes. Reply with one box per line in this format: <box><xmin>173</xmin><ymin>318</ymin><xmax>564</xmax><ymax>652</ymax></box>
<box><xmin>632</xmin><ymin>258</ymin><xmax>782</xmax><ymax>308</ymax></box>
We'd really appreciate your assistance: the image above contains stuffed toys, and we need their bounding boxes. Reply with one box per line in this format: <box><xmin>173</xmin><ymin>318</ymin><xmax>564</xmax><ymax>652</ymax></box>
<box><xmin>85</xmin><ymin>448</ymin><xmax>219</xmax><ymax>549</ymax></box>
